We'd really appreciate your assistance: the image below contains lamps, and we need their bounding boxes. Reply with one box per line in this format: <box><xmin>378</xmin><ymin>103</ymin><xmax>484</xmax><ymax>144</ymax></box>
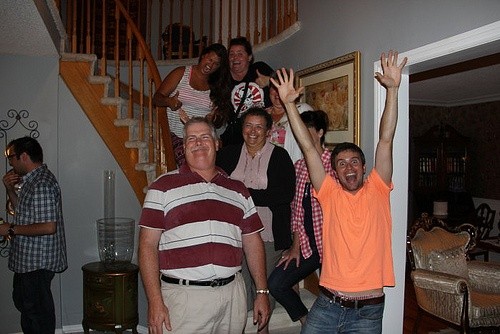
<box><xmin>433</xmin><ymin>202</ymin><xmax>447</xmax><ymax>222</ymax></box>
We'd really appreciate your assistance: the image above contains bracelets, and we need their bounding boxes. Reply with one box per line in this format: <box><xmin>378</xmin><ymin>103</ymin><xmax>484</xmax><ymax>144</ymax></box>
<box><xmin>8</xmin><ymin>224</ymin><xmax>15</xmax><ymax>237</ymax></box>
<box><xmin>255</xmin><ymin>288</ymin><xmax>270</xmax><ymax>295</ymax></box>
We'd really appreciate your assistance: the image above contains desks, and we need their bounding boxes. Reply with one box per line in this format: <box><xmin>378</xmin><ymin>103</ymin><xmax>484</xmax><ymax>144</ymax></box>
<box><xmin>82</xmin><ymin>261</ymin><xmax>140</xmax><ymax>334</ymax></box>
<box><xmin>480</xmin><ymin>239</ymin><xmax>500</xmax><ymax>262</ymax></box>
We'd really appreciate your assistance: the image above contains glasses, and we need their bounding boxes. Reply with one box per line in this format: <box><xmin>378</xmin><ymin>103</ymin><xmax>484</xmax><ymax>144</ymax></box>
<box><xmin>6</xmin><ymin>153</ymin><xmax>18</xmax><ymax>161</ymax></box>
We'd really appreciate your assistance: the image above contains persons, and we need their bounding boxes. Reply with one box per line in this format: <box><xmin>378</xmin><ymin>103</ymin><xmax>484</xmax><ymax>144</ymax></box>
<box><xmin>210</xmin><ymin>36</ymin><xmax>280</xmax><ymax>176</ymax></box>
<box><xmin>256</xmin><ymin>68</ymin><xmax>325</xmax><ymax>163</ymax></box>
<box><xmin>269</xmin><ymin>108</ymin><xmax>341</xmax><ymax>328</ymax></box>
<box><xmin>150</xmin><ymin>43</ymin><xmax>228</xmax><ymax>169</ymax></box>
<box><xmin>0</xmin><ymin>137</ymin><xmax>68</xmax><ymax>334</ymax></box>
<box><xmin>131</xmin><ymin>118</ymin><xmax>272</xmax><ymax>334</ymax></box>
<box><xmin>217</xmin><ymin>106</ymin><xmax>296</xmax><ymax>315</ymax></box>
<box><xmin>267</xmin><ymin>51</ymin><xmax>410</xmax><ymax>334</ymax></box>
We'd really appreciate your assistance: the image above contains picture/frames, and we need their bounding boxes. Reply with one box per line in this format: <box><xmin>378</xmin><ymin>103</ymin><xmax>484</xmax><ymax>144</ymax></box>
<box><xmin>295</xmin><ymin>51</ymin><xmax>361</xmax><ymax>152</ymax></box>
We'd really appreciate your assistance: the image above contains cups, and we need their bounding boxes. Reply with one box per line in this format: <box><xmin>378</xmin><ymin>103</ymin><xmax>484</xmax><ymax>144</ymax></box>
<box><xmin>96</xmin><ymin>217</ymin><xmax>135</xmax><ymax>270</ymax></box>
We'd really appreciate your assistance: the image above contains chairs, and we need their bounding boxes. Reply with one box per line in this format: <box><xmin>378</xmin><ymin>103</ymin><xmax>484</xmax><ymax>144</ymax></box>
<box><xmin>468</xmin><ymin>203</ymin><xmax>496</xmax><ymax>262</ymax></box>
<box><xmin>161</xmin><ymin>22</ymin><xmax>207</xmax><ymax>60</ymax></box>
<box><xmin>406</xmin><ymin>213</ymin><xmax>500</xmax><ymax>334</ymax></box>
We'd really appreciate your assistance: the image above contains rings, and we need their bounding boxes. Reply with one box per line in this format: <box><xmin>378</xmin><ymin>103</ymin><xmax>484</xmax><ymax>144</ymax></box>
<box><xmin>281</xmin><ymin>256</ymin><xmax>284</xmax><ymax>259</ymax></box>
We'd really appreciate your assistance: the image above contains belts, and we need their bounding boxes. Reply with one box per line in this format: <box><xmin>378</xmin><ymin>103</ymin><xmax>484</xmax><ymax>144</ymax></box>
<box><xmin>161</xmin><ymin>270</ymin><xmax>242</xmax><ymax>287</ymax></box>
<box><xmin>321</xmin><ymin>286</ymin><xmax>385</xmax><ymax>308</ymax></box>
